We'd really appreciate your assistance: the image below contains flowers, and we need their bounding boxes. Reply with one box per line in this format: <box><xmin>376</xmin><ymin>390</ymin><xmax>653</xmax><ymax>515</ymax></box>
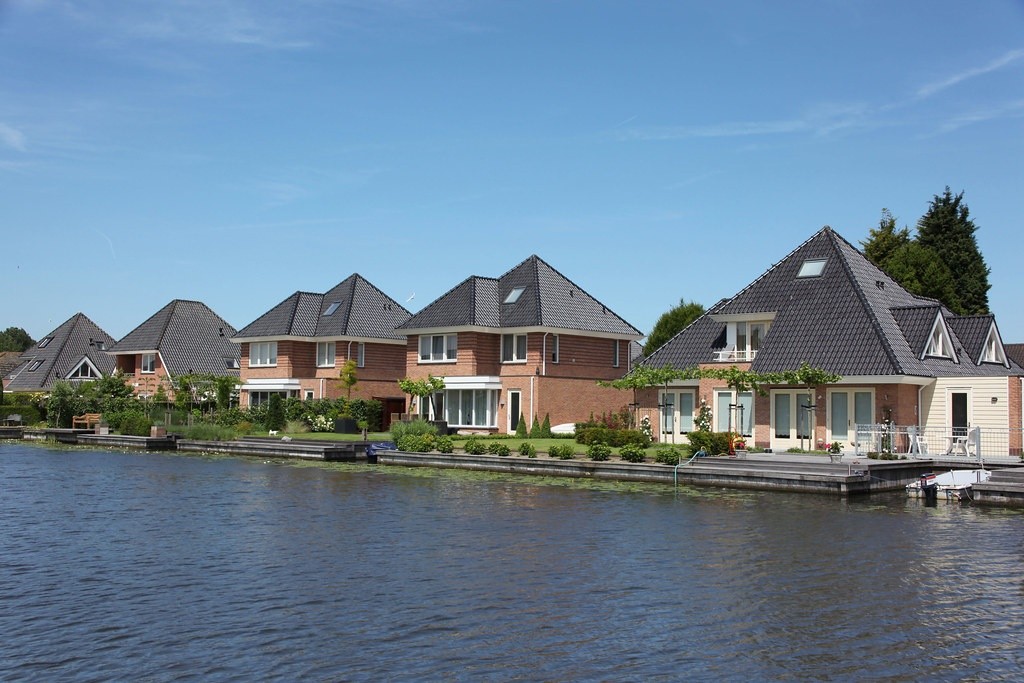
<box><xmin>735</xmin><ymin>439</ymin><xmax>747</xmax><ymax>450</ymax></box>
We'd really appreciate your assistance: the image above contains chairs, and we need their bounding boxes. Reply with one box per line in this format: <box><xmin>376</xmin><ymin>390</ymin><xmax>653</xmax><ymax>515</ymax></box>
<box><xmin>391</xmin><ymin>413</ymin><xmax>418</xmax><ymax>424</ymax></box>
<box><xmin>907</xmin><ymin>427</ymin><xmax>930</xmax><ymax>455</ymax></box>
<box><xmin>954</xmin><ymin>429</ymin><xmax>977</xmax><ymax>457</ymax></box>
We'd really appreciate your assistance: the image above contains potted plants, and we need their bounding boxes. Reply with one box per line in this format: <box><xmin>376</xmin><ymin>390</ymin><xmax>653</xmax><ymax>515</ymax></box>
<box><xmin>150</xmin><ymin>420</ymin><xmax>167</xmax><ymax>437</ymax></box>
<box><xmin>828</xmin><ymin>442</ymin><xmax>845</xmax><ymax>464</ymax></box>
<box><xmin>335</xmin><ymin>414</ymin><xmax>356</xmax><ymax>433</ymax></box>
<box><xmin>95</xmin><ymin>418</ymin><xmax>110</xmax><ymax>435</ymax></box>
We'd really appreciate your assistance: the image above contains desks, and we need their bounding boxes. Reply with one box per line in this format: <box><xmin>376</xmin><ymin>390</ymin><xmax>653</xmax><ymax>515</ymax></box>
<box><xmin>939</xmin><ymin>436</ymin><xmax>976</xmax><ymax>457</ymax></box>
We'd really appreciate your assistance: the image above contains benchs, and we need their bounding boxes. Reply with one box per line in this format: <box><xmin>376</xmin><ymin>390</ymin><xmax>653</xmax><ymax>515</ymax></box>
<box><xmin>2</xmin><ymin>414</ymin><xmax>22</xmax><ymax>426</ymax></box>
<box><xmin>72</xmin><ymin>412</ymin><xmax>102</xmax><ymax>429</ymax></box>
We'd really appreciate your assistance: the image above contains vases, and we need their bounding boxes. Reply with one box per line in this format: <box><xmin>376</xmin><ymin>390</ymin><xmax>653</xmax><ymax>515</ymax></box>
<box><xmin>735</xmin><ymin>451</ymin><xmax>747</xmax><ymax>458</ymax></box>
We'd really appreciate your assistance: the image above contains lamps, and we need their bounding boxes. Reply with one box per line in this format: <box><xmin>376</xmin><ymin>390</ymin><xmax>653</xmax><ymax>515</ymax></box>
<box><xmin>500</xmin><ymin>400</ymin><xmax>504</xmax><ymax>405</ymax></box>
